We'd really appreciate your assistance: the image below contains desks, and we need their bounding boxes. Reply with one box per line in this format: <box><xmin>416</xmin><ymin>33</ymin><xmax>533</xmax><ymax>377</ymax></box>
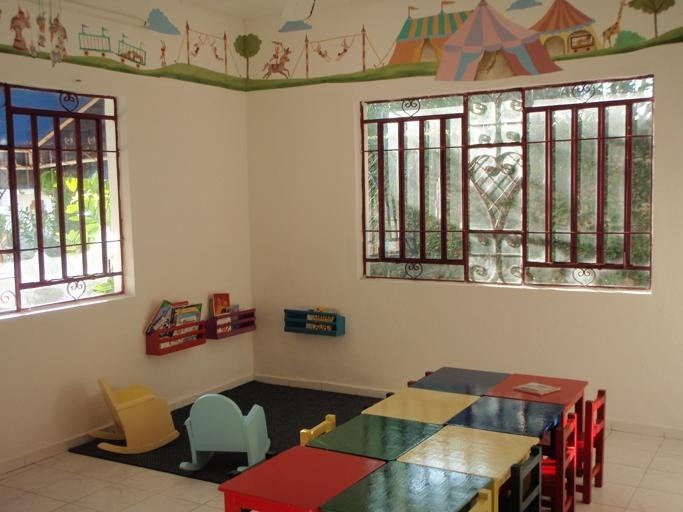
<box><xmin>218</xmin><ymin>367</ymin><xmax>588</xmax><ymax>512</ymax></box>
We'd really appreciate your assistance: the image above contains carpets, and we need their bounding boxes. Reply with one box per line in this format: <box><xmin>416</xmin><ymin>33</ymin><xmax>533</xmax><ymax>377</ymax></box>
<box><xmin>68</xmin><ymin>380</ymin><xmax>387</xmax><ymax>484</ymax></box>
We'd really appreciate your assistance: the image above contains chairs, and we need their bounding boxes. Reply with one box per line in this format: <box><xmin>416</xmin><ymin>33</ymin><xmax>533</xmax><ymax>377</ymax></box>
<box><xmin>576</xmin><ymin>389</ymin><xmax>605</xmax><ymax>504</ymax></box>
<box><xmin>88</xmin><ymin>378</ymin><xmax>180</xmax><ymax>455</ymax></box>
<box><xmin>178</xmin><ymin>393</ymin><xmax>271</xmax><ymax>472</ymax></box>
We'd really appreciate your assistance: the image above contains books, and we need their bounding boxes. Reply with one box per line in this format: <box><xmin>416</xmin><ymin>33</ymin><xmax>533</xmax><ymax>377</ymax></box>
<box><xmin>144</xmin><ymin>293</ymin><xmax>240</xmax><ymax>348</ymax></box>
<box><xmin>513</xmin><ymin>383</ymin><xmax>560</xmax><ymax>395</ymax></box>
